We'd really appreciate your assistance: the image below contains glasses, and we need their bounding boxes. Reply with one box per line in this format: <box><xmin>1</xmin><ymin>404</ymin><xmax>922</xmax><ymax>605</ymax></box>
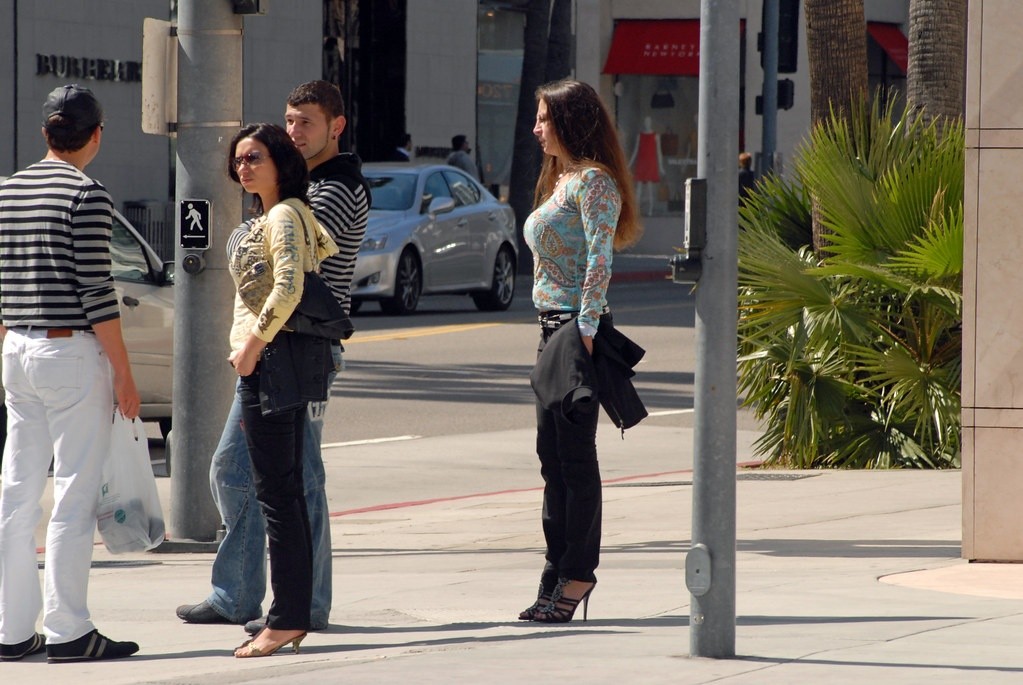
<box><xmin>98</xmin><ymin>121</ymin><xmax>104</xmax><ymax>130</ymax></box>
<box><xmin>232</xmin><ymin>152</ymin><xmax>270</xmax><ymax>170</ymax></box>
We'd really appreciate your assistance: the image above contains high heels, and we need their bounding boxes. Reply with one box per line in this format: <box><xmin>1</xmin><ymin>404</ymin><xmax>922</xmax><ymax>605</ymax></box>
<box><xmin>232</xmin><ymin>632</ymin><xmax>307</xmax><ymax>657</ymax></box>
<box><xmin>518</xmin><ymin>578</ymin><xmax>558</xmax><ymax>620</ymax></box>
<box><xmin>534</xmin><ymin>578</ymin><xmax>596</xmax><ymax>624</ymax></box>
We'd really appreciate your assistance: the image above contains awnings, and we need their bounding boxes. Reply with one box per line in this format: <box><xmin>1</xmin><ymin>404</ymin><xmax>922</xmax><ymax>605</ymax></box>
<box><xmin>867</xmin><ymin>21</ymin><xmax>908</xmax><ymax>72</ymax></box>
<box><xmin>600</xmin><ymin>19</ymin><xmax>743</xmax><ymax>77</ymax></box>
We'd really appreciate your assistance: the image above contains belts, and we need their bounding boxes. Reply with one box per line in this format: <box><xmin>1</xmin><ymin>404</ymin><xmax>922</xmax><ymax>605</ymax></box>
<box><xmin>538</xmin><ymin>306</ymin><xmax>610</xmax><ymax>329</ymax></box>
<box><xmin>16</xmin><ymin>325</ymin><xmax>96</xmax><ymax>335</ymax></box>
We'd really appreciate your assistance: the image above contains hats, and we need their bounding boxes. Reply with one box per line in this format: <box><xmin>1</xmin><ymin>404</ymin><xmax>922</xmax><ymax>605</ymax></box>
<box><xmin>42</xmin><ymin>84</ymin><xmax>101</xmax><ymax>133</ymax></box>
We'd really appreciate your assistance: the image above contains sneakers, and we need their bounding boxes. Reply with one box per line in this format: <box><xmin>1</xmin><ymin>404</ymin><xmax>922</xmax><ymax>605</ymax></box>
<box><xmin>46</xmin><ymin>628</ymin><xmax>140</xmax><ymax>663</ymax></box>
<box><xmin>0</xmin><ymin>632</ymin><xmax>46</xmax><ymax>661</ymax></box>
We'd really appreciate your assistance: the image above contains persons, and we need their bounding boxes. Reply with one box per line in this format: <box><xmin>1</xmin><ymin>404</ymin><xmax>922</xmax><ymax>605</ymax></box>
<box><xmin>393</xmin><ymin>132</ymin><xmax>412</xmax><ymax>161</ymax></box>
<box><xmin>0</xmin><ymin>83</ymin><xmax>141</xmax><ymax>665</ymax></box>
<box><xmin>522</xmin><ymin>79</ymin><xmax>643</xmax><ymax>624</ymax></box>
<box><xmin>446</xmin><ymin>135</ymin><xmax>480</xmax><ymax>183</ymax></box>
<box><xmin>224</xmin><ymin>121</ymin><xmax>339</xmax><ymax>657</ymax></box>
<box><xmin>737</xmin><ymin>152</ymin><xmax>754</xmax><ymax>212</ymax></box>
<box><xmin>625</xmin><ymin>116</ymin><xmax>665</xmax><ymax>217</ymax></box>
<box><xmin>177</xmin><ymin>78</ymin><xmax>374</xmax><ymax>633</ymax></box>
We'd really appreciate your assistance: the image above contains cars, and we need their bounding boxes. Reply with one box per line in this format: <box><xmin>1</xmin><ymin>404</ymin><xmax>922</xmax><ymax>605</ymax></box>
<box><xmin>348</xmin><ymin>160</ymin><xmax>521</xmax><ymax>317</ymax></box>
<box><xmin>0</xmin><ymin>175</ymin><xmax>175</xmax><ymax>474</ymax></box>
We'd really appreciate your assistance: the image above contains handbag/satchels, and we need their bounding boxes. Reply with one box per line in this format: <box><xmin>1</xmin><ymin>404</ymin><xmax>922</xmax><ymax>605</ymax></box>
<box><xmin>96</xmin><ymin>406</ymin><xmax>165</xmax><ymax>554</ymax></box>
<box><xmin>282</xmin><ymin>270</ymin><xmax>354</xmax><ymax>341</ymax></box>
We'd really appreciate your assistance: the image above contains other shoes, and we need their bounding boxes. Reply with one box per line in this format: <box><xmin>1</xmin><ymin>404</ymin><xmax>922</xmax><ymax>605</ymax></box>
<box><xmin>175</xmin><ymin>601</ymin><xmax>247</xmax><ymax>626</ymax></box>
<box><xmin>244</xmin><ymin>616</ymin><xmax>314</xmax><ymax>632</ymax></box>
